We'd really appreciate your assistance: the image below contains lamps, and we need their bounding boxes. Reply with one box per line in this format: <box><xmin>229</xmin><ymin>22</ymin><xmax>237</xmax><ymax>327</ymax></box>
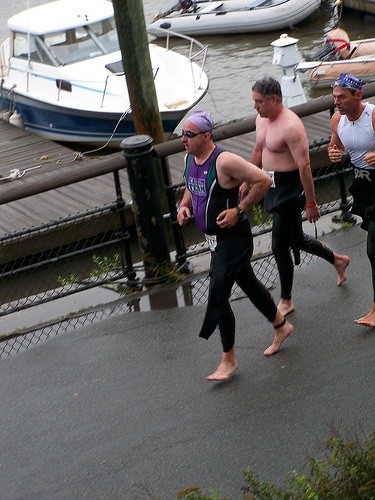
<box><xmin>270</xmin><ymin>33</ymin><xmax>302</xmax><ymax>80</ymax></box>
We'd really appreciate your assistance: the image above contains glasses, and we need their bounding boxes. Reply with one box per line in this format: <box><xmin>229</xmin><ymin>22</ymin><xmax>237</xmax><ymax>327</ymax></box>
<box><xmin>331</xmin><ymin>81</ymin><xmax>355</xmax><ymax>91</ymax></box>
<box><xmin>181</xmin><ymin>130</ymin><xmax>202</xmax><ymax>138</ymax></box>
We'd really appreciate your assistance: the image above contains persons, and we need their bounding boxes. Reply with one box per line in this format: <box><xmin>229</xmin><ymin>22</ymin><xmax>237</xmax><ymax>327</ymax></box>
<box><xmin>175</xmin><ymin>111</ymin><xmax>294</xmax><ymax>380</ymax></box>
<box><xmin>239</xmin><ymin>77</ymin><xmax>349</xmax><ymax>314</ymax></box>
<box><xmin>327</xmin><ymin>73</ymin><xmax>375</xmax><ymax>327</ymax></box>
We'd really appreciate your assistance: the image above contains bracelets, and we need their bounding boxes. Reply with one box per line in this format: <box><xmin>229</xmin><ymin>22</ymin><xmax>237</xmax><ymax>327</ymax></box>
<box><xmin>306</xmin><ymin>201</ymin><xmax>316</xmax><ymax>208</ymax></box>
<box><xmin>177</xmin><ymin>203</ymin><xmax>188</xmax><ymax>212</ymax></box>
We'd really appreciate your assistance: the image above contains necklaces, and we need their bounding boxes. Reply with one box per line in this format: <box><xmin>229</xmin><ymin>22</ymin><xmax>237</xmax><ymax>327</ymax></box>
<box><xmin>348</xmin><ymin>109</ymin><xmax>364</xmax><ymax>121</ymax></box>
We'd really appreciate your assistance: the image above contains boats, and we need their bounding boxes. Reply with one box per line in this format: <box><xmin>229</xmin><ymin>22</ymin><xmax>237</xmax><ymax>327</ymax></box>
<box><xmin>146</xmin><ymin>0</ymin><xmax>326</xmax><ymax>37</ymax></box>
<box><xmin>295</xmin><ymin>37</ymin><xmax>375</xmax><ymax>90</ymax></box>
<box><xmin>1</xmin><ymin>0</ymin><xmax>211</xmax><ymax>152</ymax></box>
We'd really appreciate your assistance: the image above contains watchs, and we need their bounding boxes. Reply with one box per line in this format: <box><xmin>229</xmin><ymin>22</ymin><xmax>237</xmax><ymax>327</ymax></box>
<box><xmin>236</xmin><ymin>207</ymin><xmax>244</xmax><ymax>218</ymax></box>
